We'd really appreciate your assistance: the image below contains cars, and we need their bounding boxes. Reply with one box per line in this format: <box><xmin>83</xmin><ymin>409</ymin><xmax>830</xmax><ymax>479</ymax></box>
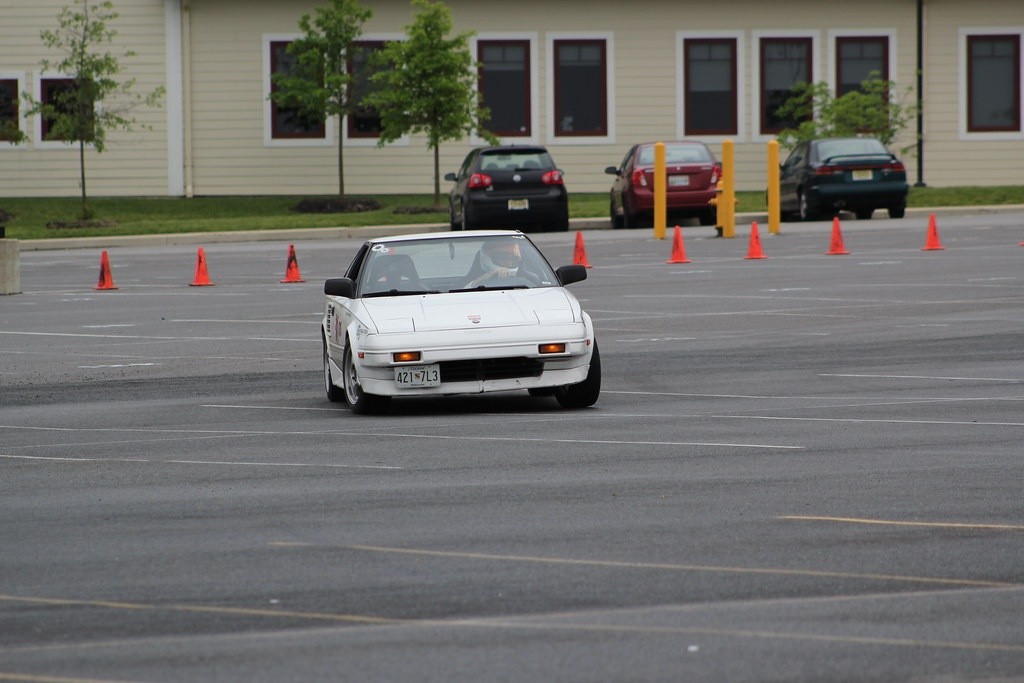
<box><xmin>766</xmin><ymin>137</ymin><xmax>909</xmax><ymax>221</ymax></box>
<box><xmin>444</xmin><ymin>143</ymin><xmax>569</xmax><ymax>232</ymax></box>
<box><xmin>321</xmin><ymin>231</ymin><xmax>601</xmax><ymax>413</ymax></box>
<box><xmin>605</xmin><ymin>142</ymin><xmax>722</xmax><ymax>228</ymax></box>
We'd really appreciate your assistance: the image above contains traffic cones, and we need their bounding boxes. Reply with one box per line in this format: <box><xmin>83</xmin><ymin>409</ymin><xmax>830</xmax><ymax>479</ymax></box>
<box><xmin>189</xmin><ymin>247</ymin><xmax>215</xmax><ymax>286</ymax></box>
<box><xmin>280</xmin><ymin>244</ymin><xmax>306</xmax><ymax>283</ymax></box>
<box><xmin>825</xmin><ymin>217</ymin><xmax>849</xmax><ymax>254</ymax></box>
<box><xmin>94</xmin><ymin>250</ymin><xmax>118</xmax><ymax>290</ymax></box>
<box><xmin>572</xmin><ymin>231</ymin><xmax>592</xmax><ymax>268</ymax></box>
<box><xmin>921</xmin><ymin>214</ymin><xmax>944</xmax><ymax>250</ymax></box>
<box><xmin>666</xmin><ymin>226</ymin><xmax>692</xmax><ymax>264</ymax></box>
<box><xmin>383</xmin><ymin>248</ymin><xmax>396</xmax><ymax>281</ymax></box>
<box><xmin>743</xmin><ymin>221</ymin><xmax>768</xmax><ymax>259</ymax></box>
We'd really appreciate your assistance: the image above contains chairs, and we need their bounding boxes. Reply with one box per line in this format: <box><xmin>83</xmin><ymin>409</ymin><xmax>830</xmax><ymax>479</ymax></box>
<box><xmin>524</xmin><ymin>160</ymin><xmax>540</xmax><ymax>168</ymax></box>
<box><xmin>455</xmin><ymin>249</ymin><xmax>540</xmax><ymax>290</ymax></box>
<box><xmin>365</xmin><ymin>253</ymin><xmax>428</xmax><ymax>296</ymax></box>
<box><xmin>485</xmin><ymin>163</ymin><xmax>497</xmax><ymax>170</ymax></box>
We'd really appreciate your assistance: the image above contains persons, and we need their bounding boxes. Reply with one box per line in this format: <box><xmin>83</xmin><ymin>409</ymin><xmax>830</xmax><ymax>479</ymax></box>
<box><xmin>462</xmin><ymin>238</ymin><xmax>539</xmax><ymax>288</ymax></box>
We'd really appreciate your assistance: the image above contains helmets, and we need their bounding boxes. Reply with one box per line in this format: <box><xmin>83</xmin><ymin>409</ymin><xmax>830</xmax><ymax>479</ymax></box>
<box><xmin>479</xmin><ymin>239</ymin><xmax>522</xmax><ymax>277</ymax></box>
